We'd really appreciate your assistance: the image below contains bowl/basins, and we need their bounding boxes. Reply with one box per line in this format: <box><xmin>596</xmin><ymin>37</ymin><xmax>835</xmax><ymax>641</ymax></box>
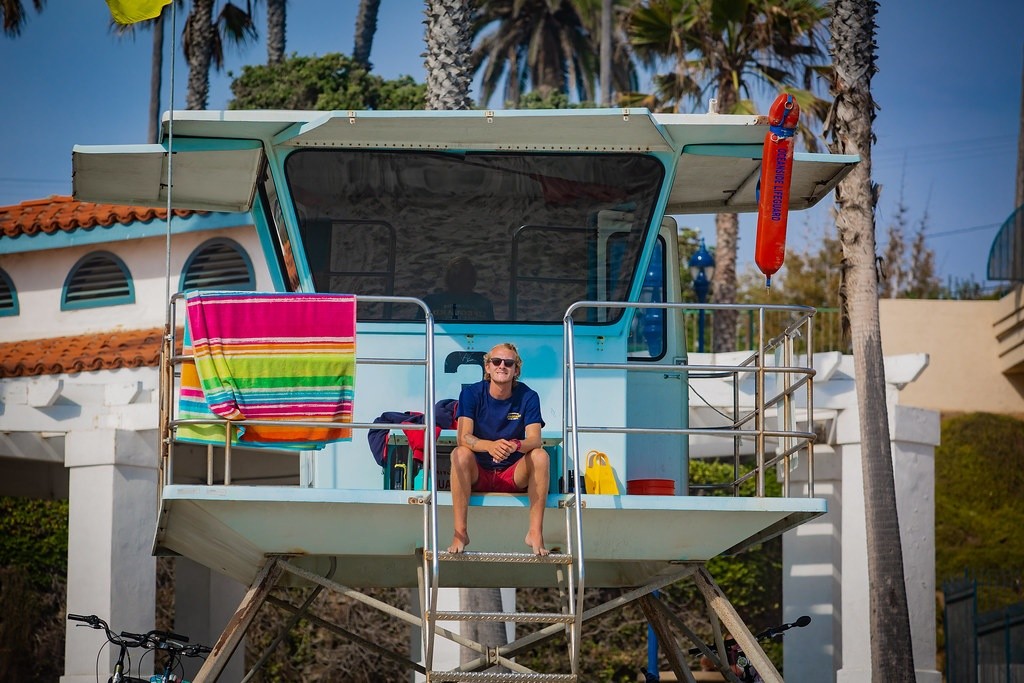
<box><xmin>628</xmin><ymin>479</ymin><xmax>675</xmax><ymax>495</ymax></box>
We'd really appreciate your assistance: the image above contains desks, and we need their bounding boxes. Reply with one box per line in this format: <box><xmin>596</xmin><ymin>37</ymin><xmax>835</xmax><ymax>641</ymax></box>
<box><xmin>384</xmin><ymin>432</ymin><xmax>565</xmax><ymax>495</ymax></box>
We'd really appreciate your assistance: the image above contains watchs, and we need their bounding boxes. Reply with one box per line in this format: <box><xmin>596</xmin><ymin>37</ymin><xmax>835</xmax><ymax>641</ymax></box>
<box><xmin>508</xmin><ymin>439</ymin><xmax>521</xmax><ymax>451</ymax></box>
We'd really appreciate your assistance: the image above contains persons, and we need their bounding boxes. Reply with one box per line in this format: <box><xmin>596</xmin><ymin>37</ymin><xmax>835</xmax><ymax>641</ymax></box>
<box><xmin>447</xmin><ymin>342</ymin><xmax>552</xmax><ymax>556</ymax></box>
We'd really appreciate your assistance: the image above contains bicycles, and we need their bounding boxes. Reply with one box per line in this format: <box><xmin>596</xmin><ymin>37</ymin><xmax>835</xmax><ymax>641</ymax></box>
<box><xmin>68</xmin><ymin>613</ymin><xmax>211</xmax><ymax>683</ymax></box>
<box><xmin>688</xmin><ymin>615</ymin><xmax>812</xmax><ymax>683</ymax></box>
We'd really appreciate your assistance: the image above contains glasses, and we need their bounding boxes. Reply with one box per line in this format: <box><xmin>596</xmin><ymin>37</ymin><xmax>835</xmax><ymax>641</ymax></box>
<box><xmin>487</xmin><ymin>358</ymin><xmax>518</xmax><ymax>367</ymax></box>
